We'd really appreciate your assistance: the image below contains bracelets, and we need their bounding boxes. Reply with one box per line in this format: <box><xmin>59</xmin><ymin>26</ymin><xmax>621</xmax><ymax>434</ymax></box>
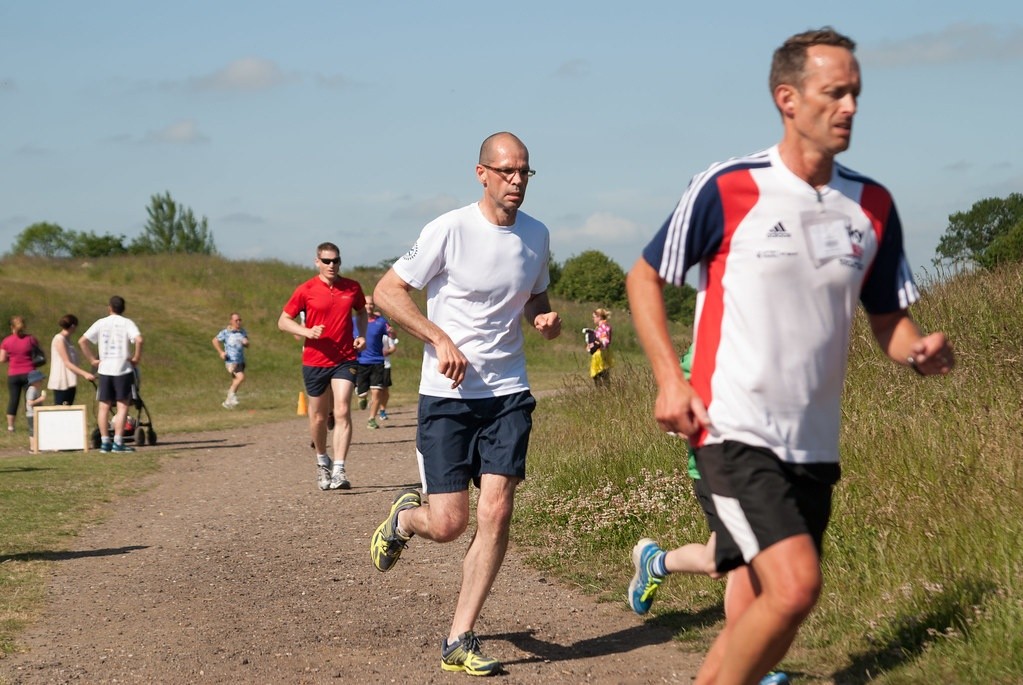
<box><xmin>908</xmin><ymin>356</ymin><xmax>925</xmax><ymax>376</ymax></box>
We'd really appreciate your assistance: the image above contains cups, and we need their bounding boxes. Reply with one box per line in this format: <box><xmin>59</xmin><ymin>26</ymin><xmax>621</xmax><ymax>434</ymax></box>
<box><xmin>41</xmin><ymin>390</ymin><xmax>46</xmax><ymax>397</ymax></box>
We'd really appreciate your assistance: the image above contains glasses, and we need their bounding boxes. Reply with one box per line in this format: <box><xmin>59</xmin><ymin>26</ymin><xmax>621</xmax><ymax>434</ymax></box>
<box><xmin>233</xmin><ymin>318</ymin><xmax>242</xmax><ymax>323</ymax></box>
<box><xmin>482</xmin><ymin>164</ymin><xmax>536</xmax><ymax>178</ymax></box>
<box><xmin>320</xmin><ymin>257</ymin><xmax>341</xmax><ymax>264</ymax></box>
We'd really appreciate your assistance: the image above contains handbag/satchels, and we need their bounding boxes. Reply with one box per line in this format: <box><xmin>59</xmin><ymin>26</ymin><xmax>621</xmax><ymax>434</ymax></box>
<box><xmin>28</xmin><ymin>336</ymin><xmax>47</xmax><ymax>366</ymax></box>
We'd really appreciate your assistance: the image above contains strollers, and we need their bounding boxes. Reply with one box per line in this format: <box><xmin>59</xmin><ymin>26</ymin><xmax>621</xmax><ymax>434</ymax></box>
<box><xmin>84</xmin><ymin>356</ymin><xmax>158</xmax><ymax>449</ymax></box>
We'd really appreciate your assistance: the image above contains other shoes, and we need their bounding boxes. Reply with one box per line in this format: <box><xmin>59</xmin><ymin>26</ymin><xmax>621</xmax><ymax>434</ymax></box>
<box><xmin>221</xmin><ymin>392</ymin><xmax>239</xmax><ymax>410</ymax></box>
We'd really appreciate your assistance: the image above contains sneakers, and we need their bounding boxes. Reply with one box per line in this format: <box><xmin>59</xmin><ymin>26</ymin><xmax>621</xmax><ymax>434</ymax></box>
<box><xmin>628</xmin><ymin>538</ymin><xmax>658</xmax><ymax>615</ymax></box>
<box><xmin>358</xmin><ymin>395</ymin><xmax>367</xmax><ymax>410</ymax></box>
<box><xmin>370</xmin><ymin>489</ymin><xmax>421</xmax><ymax>573</ymax></box>
<box><xmin>379</xmin><ymin>410</ymin><xmax>388</xmax><ymax>420</ymax></box>
<box><xmin>760</xmin><ymin>671</ymin><xmax>789</xmax><ymax>685</ymax></box>
<box><xmin>441</xmin><ymin>630</ymin><xmax>500</xmax><ymax>676</ymax></box>
<box><xmin>324</xmin><ymin>411</ymin><xmax>335</xmax><ymax>429</ymax></box>
<box><xmin>101</xmin><ymin>441</ymin><xmax>110</xmax><ymax>453</ymax></box>
<box><xmin>315</xmin><ymin>455</ymin><xmax>332</xmax><ymax>490</ymax></box>
<box><xmin>111</xmin><ymin>443</ymin><xmax>136</xmax><ymax>452</ymax></box>
<box><xmin>329</xmin><ymin>469</ymin><xmax>351</xmax><ymax>489</ymax></box>
<box><xmin>367</xmin><ymin>418</ymin><xmax>379</xmax><ymax>430</ymax></box>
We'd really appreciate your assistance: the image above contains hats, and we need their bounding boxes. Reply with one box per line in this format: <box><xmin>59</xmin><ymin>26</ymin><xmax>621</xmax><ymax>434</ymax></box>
<box><xmin>28</xmin><ymin>370</ymin><xmax>44</xmax><ymax>384</ymax></box>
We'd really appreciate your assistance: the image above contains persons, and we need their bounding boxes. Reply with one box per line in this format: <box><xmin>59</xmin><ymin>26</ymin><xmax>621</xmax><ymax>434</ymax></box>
<box><xmin>46</xmin><ymin>315</ymin><xmax>95</xmax><ymax>405</ymax></box>
<box><xmin>293</xmin><ymin>311</ymin><xmax>335</xmax><ymax>449</ymax></box>
<box><xmin>78</xmin><ymin>295</ymin><xmax>145</xmax><ymax>454</ymax></box>
<box><xmin>626</xmin><ymin>25</ymin><xmax>958</xmax><ymax>685</ymax></box>
<box><xmin>352</xmin><ymin>295</ymin><xmax>399</xmax><ymax>429</ymax></box>
<box><xmin>370</xmin><ymin>131</ymin><xmax>561</xmax><ymax>676</ymax></box>
<box><xmin>582</xmin><ymin>308</ymin><xmax>613</xmax><ymax>388</ymax></box>
<box><xmin>25</xmin><ymin>370</ymin><xmax>48</xmax><ymax>455</ymax></box>
<box><xmin>0</xmin><ymin>315</ymin><xmax>47</xmax><ymax>431</ymax></box>
<box><xmin>212</xmin><ymin>312</ymin><xmax>251</xmax><ymax>410</ymax></box>
<box><xmin>278</xmin><ymin>242</ymin><xmax>368</xmax><ymax>491</ymax></box>
